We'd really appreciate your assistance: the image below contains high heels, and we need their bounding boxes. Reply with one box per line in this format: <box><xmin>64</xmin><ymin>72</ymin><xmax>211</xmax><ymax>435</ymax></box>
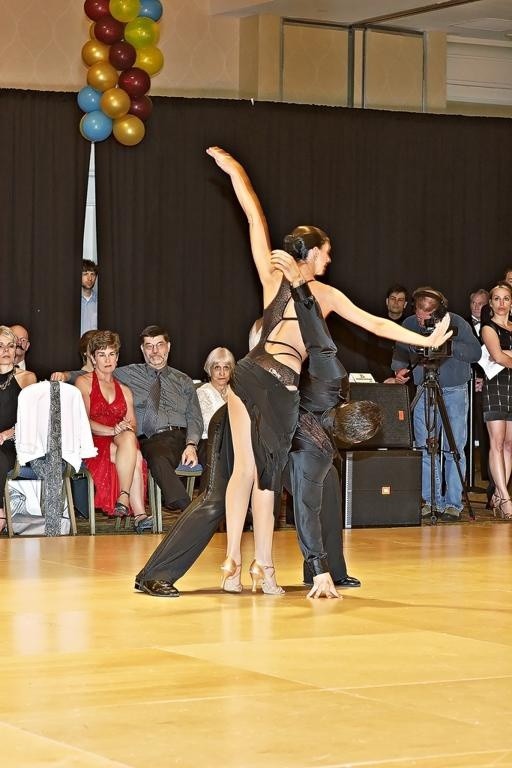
<box><xmin>490</xmin><ymin>494</ymin><xmax>512</xmax><ymax>520</ymax></box>
<box><xmin>219</xmin><ymin>557</ymin><xmax>243</xmax><ymax>593</ymax></box>
<box><xmin>115</xmin><ymin>491</ymin><xmax>129</xmax><ymax>516</ymax></box>
<box><xmin>134</xmin><ymin>514</ymin><xmax>154</xmax><ymax>534</ymax></box>
<box><xmin>249</xmin><ymin>559</ymin><xmax>285</xmax><ymax>595</ymax></box>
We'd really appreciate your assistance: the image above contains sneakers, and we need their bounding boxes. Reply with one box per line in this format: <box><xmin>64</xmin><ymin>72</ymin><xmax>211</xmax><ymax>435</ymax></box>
<box><xmin>441</xmin><ymin>507</ymin><xmax>462</xmax><ymax>521</ymax></box>
<box><xmin>420</xmin><ymin>505</ymin><xmax>444</xmax><ymax>519</ymax></box>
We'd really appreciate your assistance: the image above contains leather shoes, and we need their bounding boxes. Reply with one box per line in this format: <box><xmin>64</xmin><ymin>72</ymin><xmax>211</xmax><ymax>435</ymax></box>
<box><xmin>135</xmin><ymin>576</ymin><xmax>179</xmax><ymax>597</ymax></box>
<box><xmin>334</xmin><ymin>576</ymin><xmax>361</xmax><ymax>587</ymax></box>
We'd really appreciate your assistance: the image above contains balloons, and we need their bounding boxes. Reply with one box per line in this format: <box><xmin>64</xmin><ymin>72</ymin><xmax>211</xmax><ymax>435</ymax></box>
<box><xmin>78</xmin><ymin>0</ymin><xmax>164</xmax><ymax>146</ymax></box>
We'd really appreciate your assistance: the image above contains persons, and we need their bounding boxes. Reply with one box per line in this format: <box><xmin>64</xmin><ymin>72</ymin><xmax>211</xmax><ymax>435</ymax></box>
<box><xmin>76</xmin><ymin>258</ymin><xmax>98</xmax><ymax>338</ymax></box>
<box><xmin>205</xmin><ymin>144</ymin><xmax>454</xmax><ymax>596</ymax></box>
<box><xmin>363</xmin><ymin>269</ymin><xmax>512</xmax><ymax>521</ymax></box>
<box><xmin>67</xmin><ymin>330</ymin><xmax>153</xmax><ymax>534</ymax></box>
<box><xmin>195</xmin><ymin>347</ymin><xmax>254</xmax><ymax>533</ymax></box>
<box><xmin>1</xmin><ymin>325</ymin><xmax>37</xmax><ymax>533</ymax></box>
<box><xmin>135</xmin><ymin>248</ymin><xmax>384</xmax><ymax>602</ymax></box>
<box><xmin>50</xmin><ymin>325</ymin><xmax>208</xmax><ymax>512</ymax></box>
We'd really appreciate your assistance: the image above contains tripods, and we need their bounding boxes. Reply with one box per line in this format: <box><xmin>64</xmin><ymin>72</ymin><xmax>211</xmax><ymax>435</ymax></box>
<box><xmin>409</xmin><ymin>364</ymin><xmax>474</xmax><ymax>524</ymax></box>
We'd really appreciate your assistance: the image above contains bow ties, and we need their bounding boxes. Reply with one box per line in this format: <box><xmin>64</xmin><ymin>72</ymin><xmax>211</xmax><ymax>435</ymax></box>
<box><xmin>472</xmin><ymin>318</ymin><xmax>481</xmax><ymax>326</ymax></box>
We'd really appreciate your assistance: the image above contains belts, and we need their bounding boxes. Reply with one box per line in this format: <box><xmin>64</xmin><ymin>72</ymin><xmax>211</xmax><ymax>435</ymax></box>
<box><xmin>156</xmin><ymin>426</ymin><xmax>186</xmax><ymax>434</ymax></box>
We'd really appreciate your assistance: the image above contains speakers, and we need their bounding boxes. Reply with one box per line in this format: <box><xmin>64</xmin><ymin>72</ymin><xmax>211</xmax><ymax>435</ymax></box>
<box><xmin>341</xmin><ymin>451</ymin><xmax>422</xmax><ymax>527</ymax></box>
<box><xmin>341</xmin><ymin>383</ymin><xmax>413</xmax><ymax>448</ymax></box>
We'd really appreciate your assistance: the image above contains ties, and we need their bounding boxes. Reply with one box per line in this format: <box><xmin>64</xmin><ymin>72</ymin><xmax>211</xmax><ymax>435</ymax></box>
<box><xmin>142</xmin><ymin>371</ymin><xmax>162</xmax><ymax>439</ymax></box>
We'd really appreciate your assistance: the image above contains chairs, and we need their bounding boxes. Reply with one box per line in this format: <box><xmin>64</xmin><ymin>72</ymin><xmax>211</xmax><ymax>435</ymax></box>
<box><xmin>3</xmin><ymin>461</ymin><xmax>208</xmax><ymax>536</ymax></box>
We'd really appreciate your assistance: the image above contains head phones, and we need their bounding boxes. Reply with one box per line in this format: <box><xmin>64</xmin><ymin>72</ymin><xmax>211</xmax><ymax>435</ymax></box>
<box><xmin>409</xmin><ymin>290</ymin><xmax>447</xmax><ymax>318</ymax></box>
